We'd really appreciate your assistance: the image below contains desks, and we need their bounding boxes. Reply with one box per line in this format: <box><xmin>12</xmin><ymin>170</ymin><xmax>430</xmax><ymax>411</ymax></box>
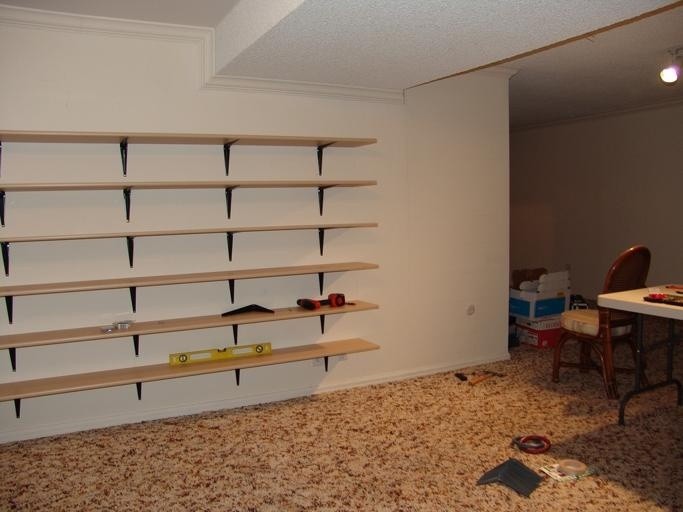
<box><xmin>594</xmin><ymin>284</ymin><xmax>683</xmax><ymax>425</ymax></box>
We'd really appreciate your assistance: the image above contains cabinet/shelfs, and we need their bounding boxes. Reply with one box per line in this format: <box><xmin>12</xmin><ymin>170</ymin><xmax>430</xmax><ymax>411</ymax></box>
<box><xmin>0</xmin><ymin>131</ymin><xmax>379</xmax><ymax>418</ymax></box>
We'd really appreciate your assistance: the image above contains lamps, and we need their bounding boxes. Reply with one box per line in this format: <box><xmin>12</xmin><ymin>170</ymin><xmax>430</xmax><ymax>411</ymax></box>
<box><xmin>659</xmin><ymin>46</ymin><xmax>683</xmax><ymax>83</ymax></box>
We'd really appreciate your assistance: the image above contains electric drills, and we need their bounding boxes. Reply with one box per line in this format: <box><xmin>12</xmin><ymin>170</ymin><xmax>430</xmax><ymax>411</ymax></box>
<box><xmin>297</xmin><ymin>293</ymin><xmax>345</xmax><ymax>311</ymax></box>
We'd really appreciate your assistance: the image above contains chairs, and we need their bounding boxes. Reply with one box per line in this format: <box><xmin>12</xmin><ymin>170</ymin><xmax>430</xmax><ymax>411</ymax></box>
<box><xmin>553</xmin><ymin>245</ymin><xmax>652</xmax><ymax>401</ymax></box>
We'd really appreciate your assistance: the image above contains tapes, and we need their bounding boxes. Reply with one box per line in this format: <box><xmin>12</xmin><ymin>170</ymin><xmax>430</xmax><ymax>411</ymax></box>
<box><xmin>559</xmin><ymin>459</ymin><xmax>587</xmax><ymax>475</ymax></box>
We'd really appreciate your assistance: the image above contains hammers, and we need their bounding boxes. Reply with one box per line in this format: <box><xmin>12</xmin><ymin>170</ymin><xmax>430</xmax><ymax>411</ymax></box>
<box><xmin>467</xmin><ymin>371</ymin><xmax>503</xmax><ymax>385</ymax></box>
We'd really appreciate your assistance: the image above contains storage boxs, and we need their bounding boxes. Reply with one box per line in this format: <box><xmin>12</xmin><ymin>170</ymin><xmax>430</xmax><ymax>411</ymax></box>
<box><xmin>509</xmin><ymin>268</ymin><xmax>570</xmax><ymax>350</ymax></box>
